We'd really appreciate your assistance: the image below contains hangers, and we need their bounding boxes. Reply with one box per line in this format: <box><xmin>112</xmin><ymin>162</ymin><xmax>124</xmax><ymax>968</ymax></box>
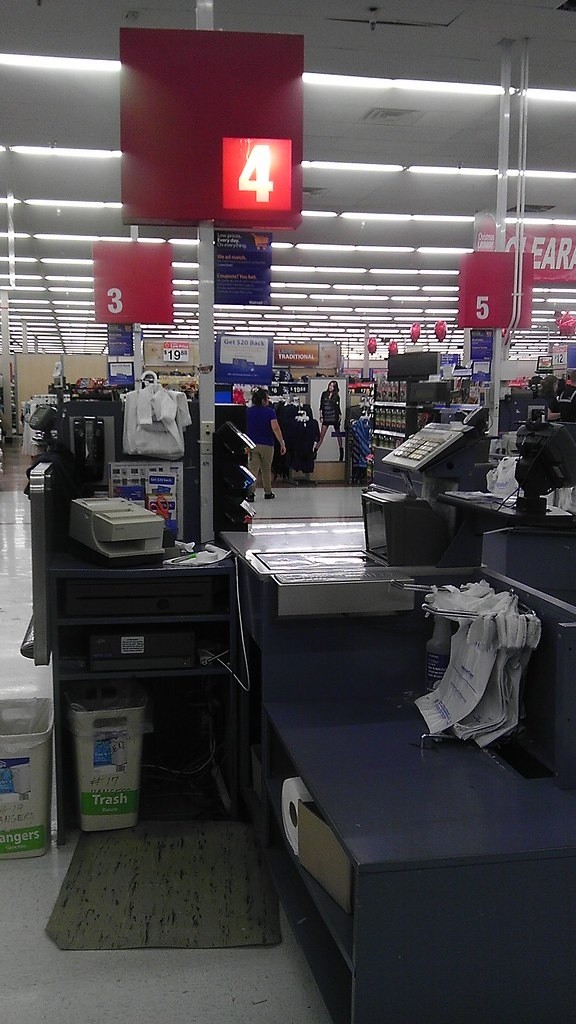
<box><xmin>123</xmin><ymin>370</ymin><xmax>181</xmax><ymax>400</ymax></box>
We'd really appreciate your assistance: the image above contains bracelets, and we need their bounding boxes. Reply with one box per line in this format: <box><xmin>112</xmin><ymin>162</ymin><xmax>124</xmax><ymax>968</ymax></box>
<box><xmin>279</xmin><ymin>440</ymin><xmax>285</xmax><ymax>444</ymax></box>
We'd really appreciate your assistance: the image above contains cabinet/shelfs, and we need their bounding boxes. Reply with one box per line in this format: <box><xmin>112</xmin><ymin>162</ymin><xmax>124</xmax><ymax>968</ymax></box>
<box><xmin>261</xmin><ymin>698</ymin><xmax>576</xmax><ymax>1024</ymax></box>
<box><xmin>373</xmin><ymin>381</ymin><xmax>451</xmax><ymax>497</ymax></box>
<box><xmin>49</xmin><ymin>549</ymin><xmax>241</xmax><ymax>825</ymax></box>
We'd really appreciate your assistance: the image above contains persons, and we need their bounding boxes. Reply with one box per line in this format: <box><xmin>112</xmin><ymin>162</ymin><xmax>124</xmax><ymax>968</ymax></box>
<box><xmin>538</xmin><ymin>375</ymin><xmax>561</xmax><ymax>422</ymax></box>
<box><xmin>312</xmin><ymin>381</ymin><xmax>344</xmax><ymax>462</ymax></box>
<box><xmin>556</xmin><ymin>370</ymin><xmax>576</xmax><ymax>423</ymax></box>
<box><xmin>246</xmin><ymin>390</ymin><xmax>286</xmax><ymax>503</ymax></box>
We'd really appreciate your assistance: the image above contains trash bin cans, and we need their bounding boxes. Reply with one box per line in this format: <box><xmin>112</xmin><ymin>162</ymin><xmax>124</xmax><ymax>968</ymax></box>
<box><xmin>0</xmin><ymin>696</ymin><xmax>55</xmax><ymax>861</ymax></box>
<box><xmin>65</xmin><ymin>693</ymin><xmax>146</xmax><ymax>833</ymax></box>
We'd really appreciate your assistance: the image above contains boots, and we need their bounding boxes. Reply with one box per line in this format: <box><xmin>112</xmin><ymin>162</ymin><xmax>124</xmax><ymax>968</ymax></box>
<box><xmin>313</xmin><ymin>448</ymin><xmax>318</xmax><ymax>459</ymax></box>
<box><xmin>339</xmin><ymin>447</ymin><xmax>344</xmax><ymax>460</ymax></box>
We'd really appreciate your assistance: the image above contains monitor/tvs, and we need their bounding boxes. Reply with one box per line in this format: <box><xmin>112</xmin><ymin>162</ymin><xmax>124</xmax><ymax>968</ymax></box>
<box><xmin>515</xmin><ymin>423</ymin><xmax>576</xmax><ymax>488</ymax></box>
<box><xmin>362</xmin><ymin>491</ymin><xmax>440</xmax><ymax>568</ymax></box>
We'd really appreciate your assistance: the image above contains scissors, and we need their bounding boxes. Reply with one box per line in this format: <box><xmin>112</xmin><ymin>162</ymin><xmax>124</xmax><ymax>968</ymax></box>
<box><xmin>148</xmin><ymin>495</ymin><xmax>174</xmax><ymax>529</ymax></box>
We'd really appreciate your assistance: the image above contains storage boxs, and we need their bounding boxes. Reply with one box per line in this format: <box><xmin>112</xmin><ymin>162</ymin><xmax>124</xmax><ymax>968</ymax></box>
<box><xmin>298</xmin><ymin>799</ymin><xmax>355</xmax><ymax>913</ymax></box>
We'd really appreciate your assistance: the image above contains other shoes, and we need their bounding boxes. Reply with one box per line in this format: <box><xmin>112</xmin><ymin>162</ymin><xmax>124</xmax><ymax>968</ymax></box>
<box><xmin>264</xmin><ymin>493</ymin><xmax>276</xmax><ymax>499</ymax></box>
<box><xmin>248</xmin><ymin>494</ymin><xmax>255</xmax><ymax>502</ymax></box>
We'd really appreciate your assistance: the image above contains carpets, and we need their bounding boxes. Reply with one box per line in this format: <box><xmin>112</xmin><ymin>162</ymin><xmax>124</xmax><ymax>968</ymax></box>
<box><xmin>45</xmin><ymin>814</ymin><xmax>284</xmax><ymax>948</ymax></box>
